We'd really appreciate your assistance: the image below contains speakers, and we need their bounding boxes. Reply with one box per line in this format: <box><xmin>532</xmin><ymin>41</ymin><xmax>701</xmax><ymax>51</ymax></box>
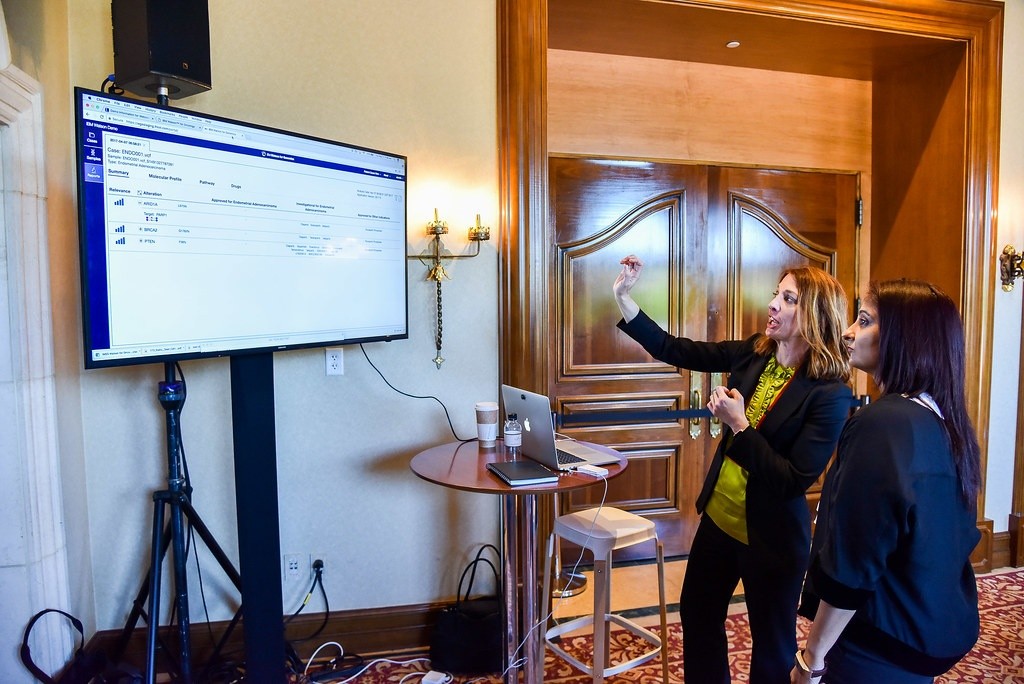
<box><xmin>111</xmin><ymin>0</ymin><xmax>212</xmax><ymax>100</ymax></box>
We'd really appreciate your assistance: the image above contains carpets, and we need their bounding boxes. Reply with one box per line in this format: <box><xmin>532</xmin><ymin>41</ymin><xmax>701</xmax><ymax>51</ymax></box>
<box><xmin>285</xmin><ymin>570</ymin><xmax>1024</xmax><ymax>684</ymax></box>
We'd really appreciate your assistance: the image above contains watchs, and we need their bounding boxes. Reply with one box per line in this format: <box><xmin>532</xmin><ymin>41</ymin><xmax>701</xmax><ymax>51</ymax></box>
<box><xmin>793</xmin><ymin>649</ymin><xmax>827</xmax><ymax>678</ymax></box>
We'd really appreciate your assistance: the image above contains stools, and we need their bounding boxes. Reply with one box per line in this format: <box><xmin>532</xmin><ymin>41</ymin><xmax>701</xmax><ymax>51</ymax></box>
<box><xmin>537</xmin><ymin>506</ymin><xmax>669</xmax><ymax>684</ymax></box>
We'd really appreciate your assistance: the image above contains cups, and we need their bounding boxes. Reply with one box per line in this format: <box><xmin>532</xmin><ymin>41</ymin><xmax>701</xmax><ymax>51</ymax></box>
<box><xmin>475</xmin><ymin>402</ymin><xmax>498</xmax><ymax>448</ymax></box>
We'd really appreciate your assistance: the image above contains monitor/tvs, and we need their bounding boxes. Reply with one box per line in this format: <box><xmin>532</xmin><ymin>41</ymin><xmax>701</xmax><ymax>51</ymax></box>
<box><xmin>73</xmin><ymin>85</ymin><xmax>409</xmax><ymax>370</ymax></box>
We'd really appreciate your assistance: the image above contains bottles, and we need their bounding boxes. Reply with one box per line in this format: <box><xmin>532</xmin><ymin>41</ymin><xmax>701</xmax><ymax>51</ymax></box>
<box><xmin>504</xmin><ymin>413</ymin><xmax>522</xmax><ymax>462</ymax></box>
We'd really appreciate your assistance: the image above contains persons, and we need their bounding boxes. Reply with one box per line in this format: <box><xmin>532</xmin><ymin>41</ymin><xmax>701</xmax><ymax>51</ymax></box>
<box><xmin>790</xmin><ymin>278</ymin><xmax>982</xmax><ymax>684</ymax></box>
<box><xmin>613</xmin><ymin>254</ymin><xmax>852</xmax><ymax>684</ymax></box>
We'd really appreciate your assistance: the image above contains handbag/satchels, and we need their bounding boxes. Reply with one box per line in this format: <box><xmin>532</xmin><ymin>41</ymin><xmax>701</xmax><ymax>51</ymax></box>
<box><xmin>429</xmin><ymin>544</ymin><xmax>523</xmax><ymax>678</ymax></box>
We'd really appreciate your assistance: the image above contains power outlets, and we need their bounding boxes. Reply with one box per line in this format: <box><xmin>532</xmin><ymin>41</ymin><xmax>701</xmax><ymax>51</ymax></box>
<box><xmin>309</xmin><ymin>553</ymin><xmax>328</xmax><ymax>581</ymax></box>
<box><xmin>325</xmin><ymin>348</ymin><xmax>343</xmax><ymax>376</ymax></box>
<box><xmin>283</xmin><ymin>553</ymin><xmax>303</xmax><ymax>580</ymax></box>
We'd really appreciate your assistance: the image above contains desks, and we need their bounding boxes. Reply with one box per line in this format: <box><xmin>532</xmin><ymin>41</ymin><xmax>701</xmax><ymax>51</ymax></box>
<box><xmin>409</xmin><ymin>438</ymin><xmax>629</xmax><ymax>683</ymax></box>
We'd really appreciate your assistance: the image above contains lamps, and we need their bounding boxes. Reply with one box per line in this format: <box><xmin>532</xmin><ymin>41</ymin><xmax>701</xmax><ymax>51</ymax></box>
<box><xmin>406</xmin><ymin>208</ymin><xmax>491</xmax><ymax>371</ymax></box>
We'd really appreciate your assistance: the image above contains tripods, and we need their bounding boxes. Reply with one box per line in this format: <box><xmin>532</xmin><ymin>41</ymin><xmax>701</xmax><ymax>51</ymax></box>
<box><xmin>101</xmin><ymin>87</ymin><xmax>306</xmax><ymax>684</ymax></box>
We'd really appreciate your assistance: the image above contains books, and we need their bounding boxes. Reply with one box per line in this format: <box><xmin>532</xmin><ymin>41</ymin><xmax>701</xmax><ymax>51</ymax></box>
<box><xmin>486</xmin><ymin>460</ymin><xmax>559</xmax><ymax>487</ymax></box>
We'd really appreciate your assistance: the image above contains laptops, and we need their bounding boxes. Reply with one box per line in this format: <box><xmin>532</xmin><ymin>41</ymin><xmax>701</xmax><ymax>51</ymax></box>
<box><xmin>501</xmin><ymin>384</ymin><xmax>621</xmax><ymax>471</ymax></box>
